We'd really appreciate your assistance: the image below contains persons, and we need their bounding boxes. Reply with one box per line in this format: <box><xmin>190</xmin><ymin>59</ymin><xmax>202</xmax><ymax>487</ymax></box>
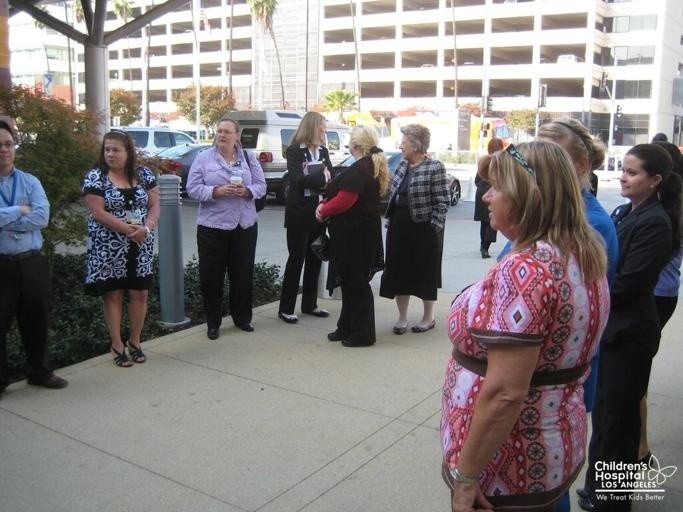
<box><xmin>635</xmin><ymin>140</ymin><xmax>683</xmax><ymax>470</ymax></box>
<box><xmin>277</xmin><ymin>111</ymin><xmax>336</xmax><ymax>323</ymax></box>
<box><xmin>439</xmin><ymin>141</ymin><xmax>612</xmax><ymax>512</ymax></box>
<box><xmin>315</xmin><ymin>123</ymin><xmax>384</xmax><ymax>348</ymax></box>
<box><xmin>473</xmin><ymin>137</ymin><xmax>505</xmax><ymax>259</ymax></box>
<box><xmin>496</xmin><ymin>117</ymin><xmax>620</xmax><ymax>512</ymax></box>
<box><xmin>0</xmin><ymin>120</ymin><xmax>68</xmax><ymax>393</ymax></box>
<box><xmin>80</xmin><ymin>130</ymin><xmax>161</xmax><ymax>368</ymax></box>
<box><xmin>185</xmin><ymin>119</ymin><xmax>268</xmax><ymax>339</ymax></box>
<box><xmin>378</xmin><ymin>124</ymin><xmax>452</xmax><ymax>334</ymax></box>
<box><xmin>576</xmin><ymin>143</ymin><xmax>674</xmax><ymax>512</ymax></box>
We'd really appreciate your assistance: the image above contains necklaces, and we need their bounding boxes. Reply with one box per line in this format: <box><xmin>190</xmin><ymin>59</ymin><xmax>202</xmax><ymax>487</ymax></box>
<box><xmin>229</xmin><ymin>151</ymin><xmax>235</xmax><ymax>166</ymax></box>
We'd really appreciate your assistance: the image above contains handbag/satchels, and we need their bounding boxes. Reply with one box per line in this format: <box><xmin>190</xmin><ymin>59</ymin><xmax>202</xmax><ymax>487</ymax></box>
<box><xmin>244</xmin><ymin>149</ymin><xmax>267</xmax><ymax>213</ymax></box>
<box><xmin>311</xmin><ymin>219</ymin><xmax>331</xmax><ymax>262</ymax></box>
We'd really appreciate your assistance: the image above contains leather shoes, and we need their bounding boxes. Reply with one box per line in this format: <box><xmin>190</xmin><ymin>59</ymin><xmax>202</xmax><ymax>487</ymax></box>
<box><xmin>236</xmin><ymin>321</ymin><xmax>253</xmax><ymax>331</ymax></box>
<box><xmin>343</xmin><ymin>337</ymin><xmax>374</xmax><ymax>346</ymax></box>
<box><xmin>640</xmin><ymin>451</ymin><xmax>653</xmax><ymax>468</ymax></box>
<box><xmin>302</xmin><ymin>308</ymin><xmax>330</xmax><ymax>317</ymax></box>
<box><xmin>577</xmin><ymin>487</ymin><xmax>588</xmax><ymax>496</ymax></box>
<box><xmin>579</xmin><ymin>496</ymin><xmax>595</xmax><ymax>510</ymax></box>
<box><xmin>279</xmin><ymin>311</ymin><xmax>297</xmax><ymax>322</ymax></box>
<box><xmin>207</xmin><ymin>327</ymin><xmax>218</xmax><ymax>339</ymax></box>
<box><xmin>328</xmin><ymin>331</ymin><xmax>348</xmax><ymax>340</ymax></box>
<box><xmin>28</xmin><ymin>374</ymin><xmax>68</xmax><ymax>389</ymax></box>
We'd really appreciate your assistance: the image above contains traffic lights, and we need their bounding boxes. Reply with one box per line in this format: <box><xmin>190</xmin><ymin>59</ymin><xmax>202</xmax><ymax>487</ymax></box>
<box><xmin>600</xmin><ymin>71</ymin><xmax>608</xmax><ymax>91</ymax></box>
<box><xmin>615</xmin><ymin>104</ymin><xmax>623</xmax><ymax>120</ymax></box>
<box><xmin>481</xmin><ymin>95</ymin><xmax>493</xmax><ymax>114</ymax></box>
<box><xmin>482</xmin><ymin>122</ymin><xmax>491</xmax><ymax>138</ymax></box>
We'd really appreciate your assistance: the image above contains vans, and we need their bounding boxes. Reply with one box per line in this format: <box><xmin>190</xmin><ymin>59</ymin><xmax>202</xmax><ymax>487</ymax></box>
<box><xmin>213</xmin><ymin>107</ymin><xmax>359</xmax><ymax>206</ymax></box>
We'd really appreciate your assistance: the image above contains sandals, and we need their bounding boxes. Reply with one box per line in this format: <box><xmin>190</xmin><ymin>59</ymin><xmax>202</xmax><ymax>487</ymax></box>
<box><xmin>412</xmin><ymin>319</ymin><xmax>435</xmax><ymax>332</ymax></box>
<box><xmin>393</xmin><ymin>321</ymin><xmax>408</xmax><ymax>333</ymax></box>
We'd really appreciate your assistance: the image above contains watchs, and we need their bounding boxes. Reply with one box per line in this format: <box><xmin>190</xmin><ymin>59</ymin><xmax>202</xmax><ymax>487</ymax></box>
<box><xmin>145</xmin><ymin>226</ymin><xmax>150</xmax><ymax>237</ymax></box>
<box><xmin>451</xmin><ymin>468</ymin><xmax>478</xmax><ymax>485</ymax></box>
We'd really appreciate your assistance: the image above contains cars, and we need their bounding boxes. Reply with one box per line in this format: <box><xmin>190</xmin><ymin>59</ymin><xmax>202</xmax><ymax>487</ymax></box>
<box><xmin>332</xmin><ymin>150</ymin><xmax>462</xmax><ymax>208</ymax></box>
<box><xmin>154</xmin><ymin>143</ymin><xmax>212</xmax><ymax>189</ymax></box>
<box><xmin>110</xmin><ymin>124</ymin><xmax>198</xmax><ymax>162</ymax></box>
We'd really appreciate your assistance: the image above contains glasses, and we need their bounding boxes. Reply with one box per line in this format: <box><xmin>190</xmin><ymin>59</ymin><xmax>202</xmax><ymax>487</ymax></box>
<box><xmin>217</xmin><ymin>129</ymin><xmax>236</xmax><ymax>134</ymax></box>
<box><xmin>0</xmin><ymin>140</ymin><xmax>15</xmax><ymax>150</ymax></box>
<box><xmin>506</xmin><ymin>144</ymin><xmax>535</xmax><ymax>177</ymax></box>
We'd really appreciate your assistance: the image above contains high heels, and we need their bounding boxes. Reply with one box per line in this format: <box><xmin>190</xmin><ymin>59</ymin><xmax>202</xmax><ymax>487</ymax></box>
<box><xmin>111</xmin><ymin>345</ymin><xmax>131</xmax><ymax>366</ymax></box>
<box><xmin>482</xmin><ymin>251</ymin><xmax>490</xmax><ymax>258</ymax></box>
<box><xmin>128</xmin><ymin>340</ymin><xmax>146</xmax><ymax>363</ymax></box>
